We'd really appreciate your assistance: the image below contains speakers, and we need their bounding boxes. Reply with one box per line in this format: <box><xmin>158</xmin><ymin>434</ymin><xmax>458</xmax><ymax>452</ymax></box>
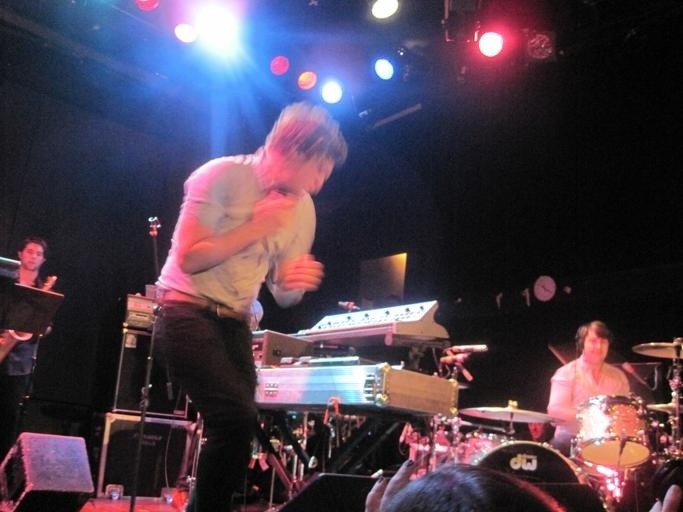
<box><xmin>276</xmin><ymin>473</ymin><xmax>391</xmax><ymax>512</ymax></box>
<box><xmin>0</xmin><ymin>432</ymin><xmax>94</xmax><ymax>512</ymax></box>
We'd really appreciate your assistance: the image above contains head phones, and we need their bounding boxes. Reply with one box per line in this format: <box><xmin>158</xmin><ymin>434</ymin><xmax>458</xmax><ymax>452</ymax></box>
<box><xmin>572</xmin><ymin>330</ymin><xmax>584</xmax><ymax>349</ymax></box>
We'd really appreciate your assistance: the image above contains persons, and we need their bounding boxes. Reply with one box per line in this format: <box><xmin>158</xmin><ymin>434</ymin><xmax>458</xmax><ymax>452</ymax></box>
<box><xmin>517</xmin><ymin>406</ymin><xmax>553</xmax><ymax>442</ymax></box>
<box><xmin>545</xmin><ymin>321</ymin><xmax>632</xmax><ymax>460</ymax></box>
<box><xmin>0</xmin><ymin>235</ymin><xmax>54</xmax><ymax>459</ymax></box>
<box><xmin>639</xmin><ymin>453</ymin><xmax>683</xmax><ymax>512</ymax></box>
<box><xmin>364</xmin><ymin>456</ymin><xmax>567</xmax><ymax>512</ymax></box>
<box><xmin>146</xmin><ymin>100</ymin><xmax>349</xmax><ymax>511</ymax></box>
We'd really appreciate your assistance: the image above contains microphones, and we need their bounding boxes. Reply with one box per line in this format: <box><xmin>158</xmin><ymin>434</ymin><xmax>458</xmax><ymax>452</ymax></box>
<box><xmin>338</xmin><ymin>301</ymin><xmax>362</xmax><ymax>311</ymax></box>
<box><xmin>439</xmin><ymin>353</ymin><xmax>472</xmax><ymax>363</ymax></box>
<box><xmin>446</xmin><ymin>350</ymin><xmax>474</xmax><ymax>382</ymax></box>
<box><xmin>452</xmin><ymin>344</ymin><xmax>489</xmax><ymax>353</ymax></box>
<box><xmin>622</xmin><ymin>362</ymin><xmax>647</xmax><ymax>386</ymax></box>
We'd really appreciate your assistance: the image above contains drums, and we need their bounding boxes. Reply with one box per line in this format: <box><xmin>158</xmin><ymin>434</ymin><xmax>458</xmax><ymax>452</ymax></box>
<box><xmin>408</xmin><ymin>432</ymin><xmax>584</xmax><ymax>482</ymax></box>
<box><xmin>574</xmin><ymin>395</ymin><xmax>651</xmax><ymax>468</ymax></box>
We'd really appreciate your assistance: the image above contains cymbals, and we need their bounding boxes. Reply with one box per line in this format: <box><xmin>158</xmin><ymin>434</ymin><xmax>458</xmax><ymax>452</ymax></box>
<box><xmin>459</xmin><ymin>406</ymin><xmax>567</xmax><ymax>423</ymax></box>
<box><xmin>632</xmin><ymin>343</ymin><xmax>683</xmax><ymax>360</ymax></box>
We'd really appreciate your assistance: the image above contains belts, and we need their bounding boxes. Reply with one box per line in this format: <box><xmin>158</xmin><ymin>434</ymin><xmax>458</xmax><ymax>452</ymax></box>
<box><xmin>157</xmin><ymin>289</ymin><xmax>251</xmax><ymax>325</ymax></box>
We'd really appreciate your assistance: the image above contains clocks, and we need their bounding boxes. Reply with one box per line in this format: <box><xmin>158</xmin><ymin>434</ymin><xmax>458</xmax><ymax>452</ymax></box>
<box><xmin>533</xmin><ymin>272</ymin><xmax>559</xmax><ymax>304</ymax></box>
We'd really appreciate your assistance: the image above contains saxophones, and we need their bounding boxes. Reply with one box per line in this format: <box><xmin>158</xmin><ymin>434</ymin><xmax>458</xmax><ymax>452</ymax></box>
<box><xmin>0</xmin><ymin>276</ymin><xmax>58</xmax><ymax>362</ymax></box>
